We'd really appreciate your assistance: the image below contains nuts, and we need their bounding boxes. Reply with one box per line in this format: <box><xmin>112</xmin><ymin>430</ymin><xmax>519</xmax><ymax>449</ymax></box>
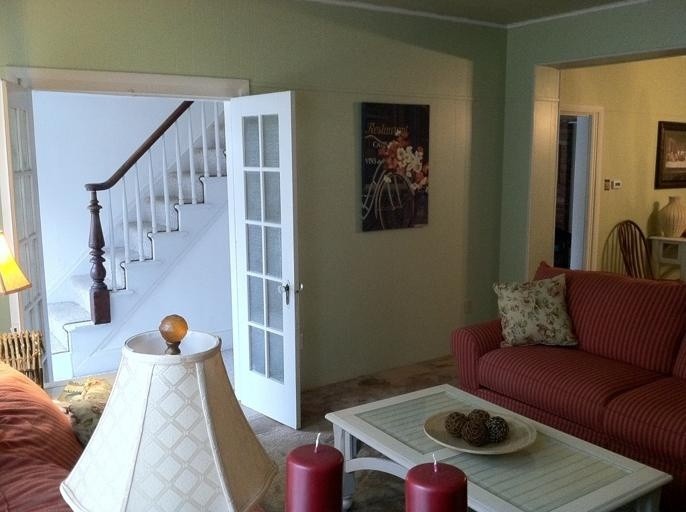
<box><xmin>445</xmin><ymin>409</ymin><xmax>509</xmax><ymax>446</ymax></box>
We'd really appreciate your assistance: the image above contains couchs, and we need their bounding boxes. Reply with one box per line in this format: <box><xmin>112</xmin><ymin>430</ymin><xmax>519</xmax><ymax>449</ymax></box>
<box><xmin>449</xmin><ymin>260</ymin><xmax>686</xmax><ymax>480</ymax></box>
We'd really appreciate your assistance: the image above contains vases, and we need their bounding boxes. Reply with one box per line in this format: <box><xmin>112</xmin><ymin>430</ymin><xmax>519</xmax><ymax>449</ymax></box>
<box><xmin>658</xmin><ymin>196</ymin><xmax>686</xmax><ymax>237</ymax></box>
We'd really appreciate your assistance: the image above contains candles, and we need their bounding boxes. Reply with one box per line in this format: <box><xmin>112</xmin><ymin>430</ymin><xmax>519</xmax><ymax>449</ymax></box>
<box><xmin>405</xmin><ymin>453</ymin><xmax>468</xmax><ymax>512</ymax></box>
<box><xmin>284</xmin><ymin>432</ymin><xmax>344</xmax><ymax>512</ymax></box>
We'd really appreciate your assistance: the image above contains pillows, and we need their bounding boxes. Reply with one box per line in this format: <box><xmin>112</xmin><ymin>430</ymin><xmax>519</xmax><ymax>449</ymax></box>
<box><xmin>491</xmin><ymin>273</ymin><xmax>578</xmax><ymax>348</ymax></box>
<box><xmin>58</xmin><ymin>375</ymin><xmax>113</xmax><ymax>448</ymax></box>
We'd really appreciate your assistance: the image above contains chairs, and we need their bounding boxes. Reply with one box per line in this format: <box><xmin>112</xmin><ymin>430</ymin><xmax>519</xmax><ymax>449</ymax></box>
<box><xmin>618</xmin><ymin>219</ymin><xmax>678</xmax><ymax>281</ymax></box>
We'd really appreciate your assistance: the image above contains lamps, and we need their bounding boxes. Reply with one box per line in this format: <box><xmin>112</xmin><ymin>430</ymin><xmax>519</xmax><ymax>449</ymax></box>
<box><xmin>59</xmin><ymin>314</ymin><xmax>279</xmax><ymax>512</ymax></box>
<box><xmin>0</xmin><ymin>231</ymin><xmax>33</xmax><ymax>295</ymax></box>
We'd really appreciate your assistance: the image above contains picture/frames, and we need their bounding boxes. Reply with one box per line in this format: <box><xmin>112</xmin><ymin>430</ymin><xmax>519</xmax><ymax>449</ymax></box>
<box><xmin>654</xmin><ymin>121</ymin><xmax>686</xmax><ymax>189</ymax></box>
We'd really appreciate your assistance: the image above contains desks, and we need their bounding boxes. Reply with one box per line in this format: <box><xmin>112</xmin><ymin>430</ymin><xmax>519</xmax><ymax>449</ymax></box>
<box><xmin>648</xmin><ymin>235</ymin><xmax>686</xmax><ymax>281</ymax></box>
<box><xmin>324</xmin><ymin>383</ymin><xmax>673</xmax><ymax>512</ymax></box>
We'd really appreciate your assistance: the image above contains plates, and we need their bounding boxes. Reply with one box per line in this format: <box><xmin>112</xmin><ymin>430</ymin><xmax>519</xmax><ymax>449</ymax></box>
<box><xmin>423</xmin><ymin>410</ymin><xmax>538</xmax><ymax>457</ymax></box>
